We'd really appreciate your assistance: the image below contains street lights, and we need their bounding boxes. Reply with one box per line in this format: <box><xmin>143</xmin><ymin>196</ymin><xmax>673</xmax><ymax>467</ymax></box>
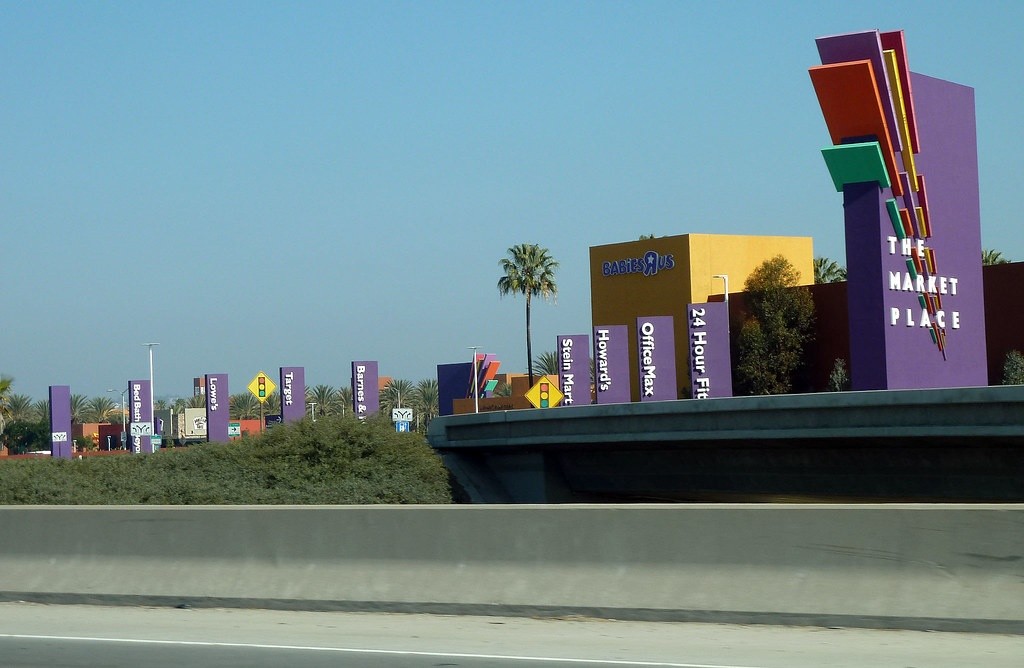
<box><xmin>140</xmin><ymin>342</ymin><xmax>159</xmax><ymax>454</ymax></box>
<box><xmin>107</xmin><ymin>388</ymin><xmax>131</xmax><ymax>450</ymax></box>
<box><xmin>713</xmin><ymin>273</ymin><xmax>730</xmax><ymax>302</ymax></box>
<box><xmin>465</xmin><ymin>345</ymin><xmax>480</xmax><ymax>413</ymax></box>
<box><xmin>308</xmin><ymin>403</ymin><xmax>319</xmax><ymax>421</ymax></box>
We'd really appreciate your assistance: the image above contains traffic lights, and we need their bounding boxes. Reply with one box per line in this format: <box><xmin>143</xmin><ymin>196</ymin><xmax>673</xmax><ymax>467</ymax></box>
<box><xmin>526</xmin><ymin>375</ymin><xmax>564</xmax><ymax>408</ymax></box>
<box><xmin>249</xmin><ymin>372</ymin><xmax>278</xmax><ymax>404</ymax></box>
